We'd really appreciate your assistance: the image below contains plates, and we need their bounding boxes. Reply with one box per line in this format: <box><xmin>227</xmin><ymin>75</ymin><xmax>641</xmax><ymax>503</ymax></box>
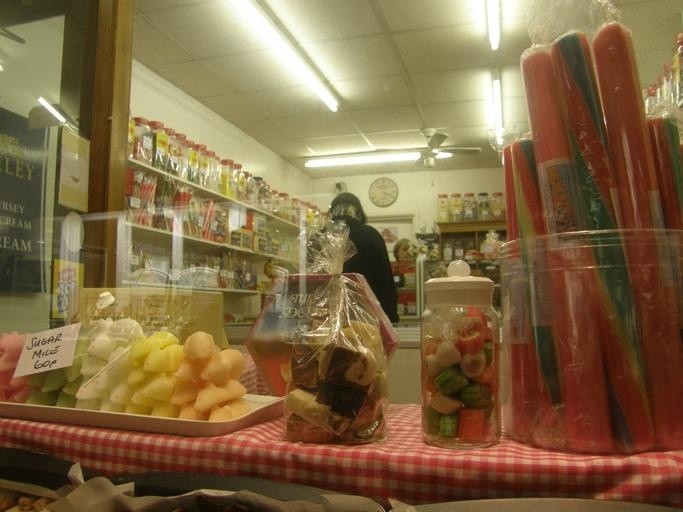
<box><xmin>0</xmin><ymin>394</ymin><xmax>287</xmax><ymax>437</ymax></box>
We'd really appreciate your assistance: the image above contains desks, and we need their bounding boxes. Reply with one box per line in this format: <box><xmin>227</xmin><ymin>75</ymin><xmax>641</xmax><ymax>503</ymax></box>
<box><xmin>0</xmin><ymin>391</ymin><xmax>683</xmax><ymax>511</ymax></box>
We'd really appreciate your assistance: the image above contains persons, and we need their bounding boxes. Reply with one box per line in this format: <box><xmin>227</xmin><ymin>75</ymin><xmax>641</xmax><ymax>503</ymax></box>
<box><xmin>305</xmin><ymin>192</ymin><xmax>399</xmax><ymax>324</ymax></box>
<box><xmin>390</xmin><ymin>237</ymin><xmax>417</xmax><ymax>274</ymax></box>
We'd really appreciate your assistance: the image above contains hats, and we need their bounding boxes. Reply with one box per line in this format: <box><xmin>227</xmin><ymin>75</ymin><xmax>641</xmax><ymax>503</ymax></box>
<box><xmin>330</xmin><ymin>192</ymin><xmax>367</xmax><ymax>223</ymax></box>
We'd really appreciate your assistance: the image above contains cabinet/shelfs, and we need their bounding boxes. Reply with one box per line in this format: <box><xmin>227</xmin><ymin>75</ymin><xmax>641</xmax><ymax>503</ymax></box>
<box><xmin>388</xmin><ymin>253</ymin><xmax>423</xmax><ymax>319</ymax></box>
<box><xmin>419</xmin><ymin>254</ymin><xmax>502</xmax><ymax>318</ymax></box>
<box><xmin>435</xmin><ymin>219</ymin><xmax>508</xmax><ymax>264</ymax></box>
<box><xmin>125</xmin><ymin>155</ymin><xmax>321</xmax><ymax>326</ymax></box>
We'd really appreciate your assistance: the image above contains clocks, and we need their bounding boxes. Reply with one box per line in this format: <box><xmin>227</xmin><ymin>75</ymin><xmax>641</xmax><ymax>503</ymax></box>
<box><xmin>368</xmin><ymin>176</ymin><xmax>399</xmax><ymax>207</ymax></box>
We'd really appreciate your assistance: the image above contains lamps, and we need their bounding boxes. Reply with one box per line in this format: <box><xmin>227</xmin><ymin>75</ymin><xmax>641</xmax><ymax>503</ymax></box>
<box><xmin>36</xmin><ymin>96</ymin><xmax>78</xmax><ymax>135</ymax></box>
<box><xmin>303</xmin><ymin>150</ymin><xmax>452</xmax><ymax>169</ymax></box>
<box><xmin>485</xmin><ymin>1</ymin><xmax>500</xmax><ymax>51</ymax></box>
<box><xmin>492</xmin><ymin>69</ymin><xmax>505</xmax><ymax>151</ymax></box>
<box><xmin>228</xmin><ymin>0</ymin><xmax>340</xmax><ymax>113</ymax></box>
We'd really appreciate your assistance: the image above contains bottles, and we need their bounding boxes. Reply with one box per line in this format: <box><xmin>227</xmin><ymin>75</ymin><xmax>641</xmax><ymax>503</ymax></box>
<box><xmin>442</xmin><ymin>243</ymin><xmax>453</xmax><ymax>261</ymax></box>
<box><xmin>148</xmin><ymin>120</ymin><xmax>169</xmax><ymax>170</ymax></box>
<box><xmin>176</xmin><ymin>133</ymin><xmax>188</xmax><ymax>181</ymax></box>
<box><xmin>208</xmin><ymin>150</ymin><xmax>219</xmax><ymax>192</ymax></box>
<box><xmin>477</xmin><ymin>192</ymin><xmax>490</xmax><ymax>221</ymax></box>
<box><xmin>165</xmin><ymin>127</ymin><xmax>180</xmax><ymax>176</ymax></box>
<box><xmin>490</xmin><ymin>192</ymin><xmax>505</xmax><ymax>221</ymax></box>
<box><xmin>463</xmin><ymin>193</ymin><xmax>477</xmax><ymax>222</ymax></box>
<box><xmin>453</xmin><ymin>241</ymin><xmax>464</xmax><ymax>259</ymax></box>
<box><xmin>221</xmin><ymin>158</ymin><xmax>234</xmax><ymax>197</ymax></box>
<box><xmin>243</xmin><ymin>170</ymin><xmax>257</xmax><ymax>205</ymax></box>
<box><xmin>449</xmin><ymin>194</ymin><xmax>463</xmax><ymax>222</ymax></box>
<box><xmin>135</xmin><ymin>117</ymin><xmax>153</xmax><ymax>166</ymax></box>
<box><xmin>233</xmin><ymin>163</ymin><xmax>246</xmax><ymax>200</ymax></box>
<box><xmin>480</xmin><ymin>230</ymin><xmax>501</xmax><ymax>261</ymax></box>
<box><xmin>186</xmin><ymin>140</ymin><xmax>198</xmax><ymax>183</ymax></box>
<box><xmin>196</xmin><ymin>144</ymin><xmax>209</xmax><ymax>186</ymax></box>
<box><xmin>436</xmin><ymin>194</ymin><xmax>449</xmax><ymax>223</ymax></box>
<box><xmin>421</xmin><ymin>259</ymin><xmax>501</xmax><ymax>449</ymax></box>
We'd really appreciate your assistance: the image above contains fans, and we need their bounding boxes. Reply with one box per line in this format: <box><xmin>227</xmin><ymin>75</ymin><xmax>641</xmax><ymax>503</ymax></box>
<box><xmin>413</xmin><ymin>128</ymin><xmax>483</xmax><ymax>169</ymax></box>
